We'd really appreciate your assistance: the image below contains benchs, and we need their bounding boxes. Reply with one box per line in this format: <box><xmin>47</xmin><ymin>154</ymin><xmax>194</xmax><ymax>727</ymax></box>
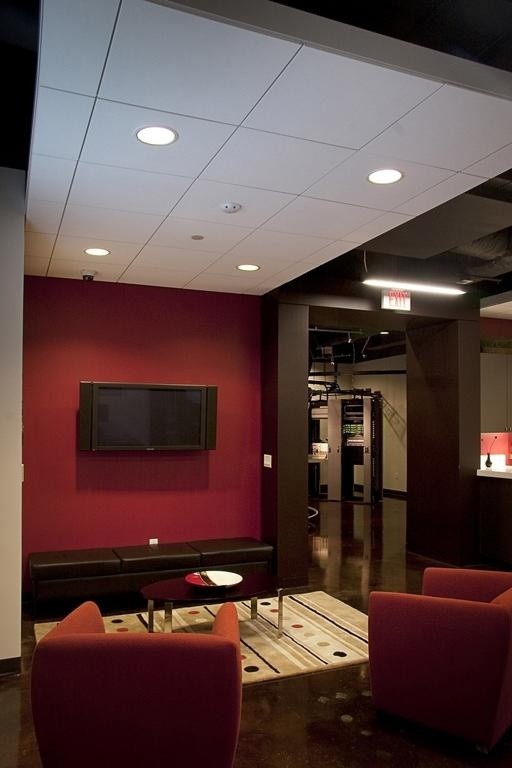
<box><xmin>26</xmin><ymin>536</ymin><xmax>275</xmax><ymax>617</ymax></box>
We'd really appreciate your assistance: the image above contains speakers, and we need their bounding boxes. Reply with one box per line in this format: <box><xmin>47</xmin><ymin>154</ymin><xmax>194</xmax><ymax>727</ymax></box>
<box><xmin>206</xmin><ymin>385</ymin><xmax>217</xmax><ymax>450</ymax></box>
<box><xmin>79</xmin><ymin>380</ymin><xmax>92</xmax><ymax>452</ymax></box>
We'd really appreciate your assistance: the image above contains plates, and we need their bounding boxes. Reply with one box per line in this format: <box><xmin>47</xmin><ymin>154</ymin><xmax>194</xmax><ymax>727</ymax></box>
<box><xmin>185</xmin><ymin>570</ymin><xmax>243</xmax><ymax>590</ymax></box>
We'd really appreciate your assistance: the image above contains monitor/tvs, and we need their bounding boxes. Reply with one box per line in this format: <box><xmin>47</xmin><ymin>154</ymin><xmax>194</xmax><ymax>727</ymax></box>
<box><xmin>92</xmin><ymin>382</ymin><xmax>206</xmax><ymax>451</ymax></box>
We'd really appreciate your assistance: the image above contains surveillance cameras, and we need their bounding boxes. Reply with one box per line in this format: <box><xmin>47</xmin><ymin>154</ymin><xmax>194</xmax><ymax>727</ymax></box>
<box><xmin>80</xmin><ymin>269</ymin><xmax>95</xmax><ymax>282</ymax></box>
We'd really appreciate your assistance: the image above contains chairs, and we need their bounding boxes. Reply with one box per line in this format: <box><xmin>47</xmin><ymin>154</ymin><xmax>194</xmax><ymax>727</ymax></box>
<box><xmin>28</xmin><ymin>601</ymin><xmax>245</xmax><ymax>768</ymax></box>
<box><xmin>366</xmin><ymin>564</ymin><xmax>512</xmax><ymax>756</ymax></box>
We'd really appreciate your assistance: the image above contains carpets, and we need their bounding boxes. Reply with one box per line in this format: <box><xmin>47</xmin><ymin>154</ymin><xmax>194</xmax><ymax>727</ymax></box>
<box><xmin>32</xmin><ymin>589</ymin><xmax>369</xmax><ymax>689</ymax></box>
